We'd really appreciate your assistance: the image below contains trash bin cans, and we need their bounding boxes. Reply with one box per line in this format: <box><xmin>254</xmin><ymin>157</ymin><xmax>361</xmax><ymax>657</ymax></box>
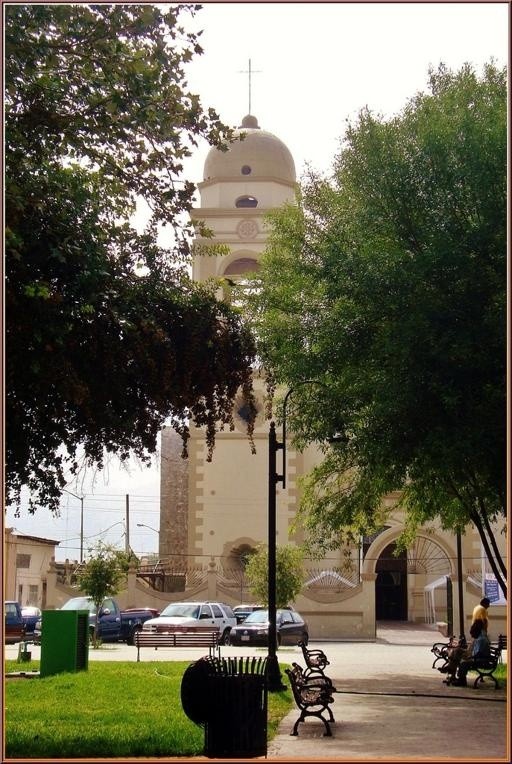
<box><xmin>181</xmin><ymin>656</ymin><xmax>268</xmax><ymax>758</ymax></box>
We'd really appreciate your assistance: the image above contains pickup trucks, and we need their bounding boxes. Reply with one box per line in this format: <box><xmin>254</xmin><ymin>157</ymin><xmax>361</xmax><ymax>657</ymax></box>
<box><xmin>5</xmin><ymin>596</ymin><xmax>152</xmax><ymax>644</ymax></box>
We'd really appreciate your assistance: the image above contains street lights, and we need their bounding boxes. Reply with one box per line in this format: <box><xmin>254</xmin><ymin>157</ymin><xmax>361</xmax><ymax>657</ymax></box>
<box><xmin>262</xmin><ymin>380</ymin><xmax>349</xmax><ymax>691</ymax></box>
<box><xmin>137</xmin><ymin>524</ymin><xmax>160</xmax><ymax>533</ymax></box>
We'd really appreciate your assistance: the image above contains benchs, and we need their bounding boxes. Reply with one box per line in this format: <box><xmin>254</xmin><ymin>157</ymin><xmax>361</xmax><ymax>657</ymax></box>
<box><xmin>285</xmin><ymin>640</ymin><xmax>338</xmax><ymax>738</ymax></box>
<box><xmin>431</xmin><ymin>632</ymin><xmax>507</xmax><ymax>690</ymax></box>
<box><xmin>133</xmin><ymin>628</ymin><xmax>219</xmax><ymax>662</ymax></box>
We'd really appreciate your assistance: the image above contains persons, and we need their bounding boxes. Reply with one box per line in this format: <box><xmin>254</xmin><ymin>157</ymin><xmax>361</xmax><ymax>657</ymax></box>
<box><xmin>437</xmin><ymin>597</ymin><xmax>490</xmax><ymax>687</ymax></box>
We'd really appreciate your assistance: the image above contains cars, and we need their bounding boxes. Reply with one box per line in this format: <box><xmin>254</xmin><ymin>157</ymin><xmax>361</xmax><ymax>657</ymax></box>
<box><xmin>142</xmin><ymin>601</ymin><xmax>308</xmax><ymax>647</ymax></box>
